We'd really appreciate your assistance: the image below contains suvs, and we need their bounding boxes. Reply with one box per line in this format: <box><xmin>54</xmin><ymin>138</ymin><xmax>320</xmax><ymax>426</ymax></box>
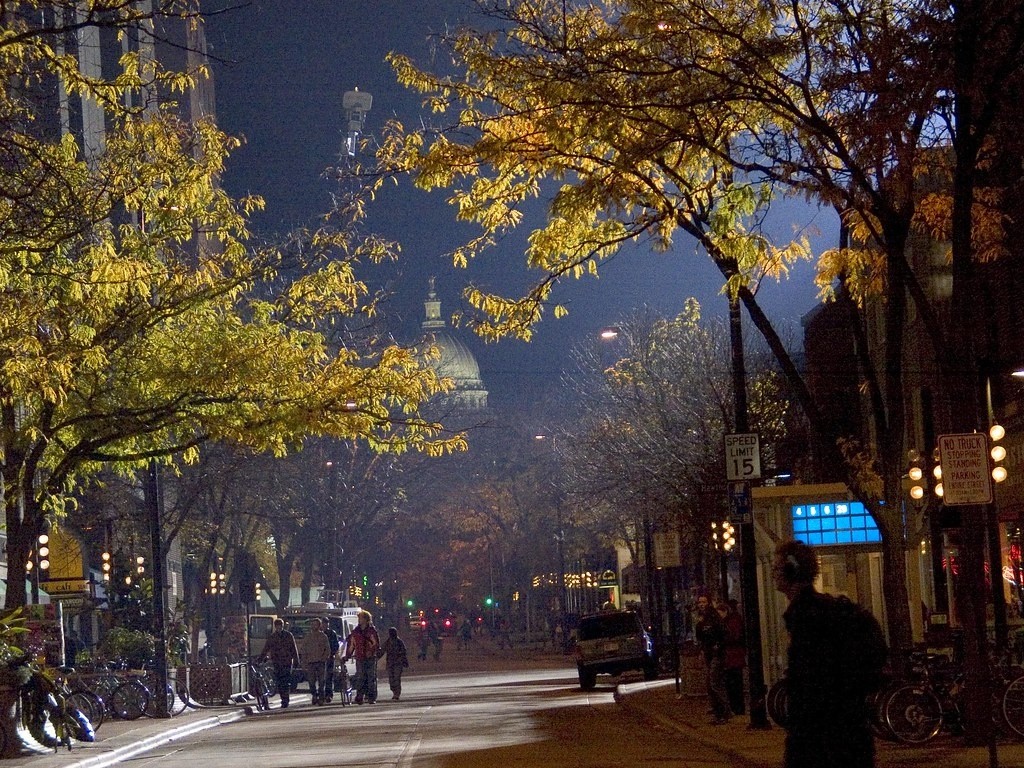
<box><xmin>421</xmin><ymin>608</ymin><xmax>458</xmax><ymax>635</ymax></box>
<box><xmin>573</xmin><ymin>607</ymin><xmax>658</xmax><ymax>688</ymax></box>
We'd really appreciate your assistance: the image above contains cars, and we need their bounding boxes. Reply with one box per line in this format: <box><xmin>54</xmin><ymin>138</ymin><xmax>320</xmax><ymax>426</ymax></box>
<box><xmin>410</xmin><ymin>616</ymin><xmax>421</xmax><ymax>629</ymax></box>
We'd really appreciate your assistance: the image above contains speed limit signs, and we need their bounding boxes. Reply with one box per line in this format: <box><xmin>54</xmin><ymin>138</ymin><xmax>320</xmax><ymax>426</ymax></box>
<box><xmin>724</xmin><ymin>431</ymin><xmax>763</xmax><ymax>482</ymax></box>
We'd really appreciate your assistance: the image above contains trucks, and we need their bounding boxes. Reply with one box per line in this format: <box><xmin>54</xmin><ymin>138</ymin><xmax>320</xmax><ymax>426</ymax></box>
<box><xmin>248</xmin><ymin>602</ymin><xmax>362</xmax><ymax>690</ymax></box>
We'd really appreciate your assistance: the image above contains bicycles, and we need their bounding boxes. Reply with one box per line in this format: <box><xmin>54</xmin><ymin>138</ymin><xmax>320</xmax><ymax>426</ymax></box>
<box><xmin>49</xmin><ymin>659</ymin><xmax>190</xmax><ymax>733</ymax></box>
<box><xmin>336</xmin><ymin>655</ymin><xmax>357</xmax><ymax>707</ymax></box>
<box><xmin>767</xmin><ymin>642</ymin><xmax>1024</xmax><ymax>744</ymax></box>
<box><xmin>240</xmin><ymin>654</ymin><xmax>278</xmax><ymax>710</ymax></box>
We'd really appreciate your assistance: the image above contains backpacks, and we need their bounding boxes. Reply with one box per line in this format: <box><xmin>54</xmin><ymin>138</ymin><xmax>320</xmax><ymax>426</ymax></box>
<box><xmin>827</xmin><ymin>596</ymin><xmax>888</xmax><ymax>698</ymax></box>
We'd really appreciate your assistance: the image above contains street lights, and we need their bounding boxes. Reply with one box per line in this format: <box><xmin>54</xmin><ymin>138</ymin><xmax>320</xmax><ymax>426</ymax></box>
<box><xmin>986</xmin><ymin>410</ymin><xmax>1009</xmax><ymax>641</ymax></box>
<box><xmin>908</xmin><ymin>445</ymin><xmax>950</xmax><ymax>638</ymax></box>
<box><xmin>103</xmin><ymin>552</ymin><xmax>116</xmax><ymax>628</ymax></box>
<box><xmin>25</xmin><ymin>534</ymin><xmax>50</xmax><ymax>605</ymax></box>
<box><xmin>712</xmin><ymin>519</ymin><xmax>735</xmax><ymax>601</ymax></box>
<box><xmin>210</xmin><ymin>572</ymin><xmax>225</xmax><ymax>628</ymax></box>
<box><xmin>253</xmin><ymin>582</ymin><xmax>261</xmax><ymax>611</ymax></box>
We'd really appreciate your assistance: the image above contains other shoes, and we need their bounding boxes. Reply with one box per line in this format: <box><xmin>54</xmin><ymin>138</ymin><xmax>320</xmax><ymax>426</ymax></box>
<box><xmin>355</xmin><ymin>696</ymin><xmax>363</xmax><ymax>705</ymax></box>
<box><xmin>369</xmin><ymin>700</ymin><xmax>376</xmax><ymax>704</ymax></box>
<box><xmin>281</xmin><ymin>699</ymin><xmax>289</xmax><ymax>708</ymax></box>
<box><xmin>319</xmin><ymin>700</ymin><xmax>323</xmax><ymax>705</ymax></box>
<box><xmin>312</xmin><ymin>697</ymin><xmax>316</xmax><ymax>705</ymax></box>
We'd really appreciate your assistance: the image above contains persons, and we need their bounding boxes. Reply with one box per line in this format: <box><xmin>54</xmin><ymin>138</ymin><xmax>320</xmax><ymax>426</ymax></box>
<box><xmin>460</xmin><ymin>620</ymin><xmax>472</xmax><ymax>651</ymax></box>
<box><xmin>381</xmin><ymin>627</ymin><xmax>409</xmax><ymax>700</ymax></box>
<box><xmin>417</xmin><ymin>627</ymin><xmax>430</xmax><ymax>660</ymax></box>
<box><xmin>342</xmin><ymin>611</ymin><xmax>380</xmax><ymax>704</ymax></box>
<box><xmin>500</xmin><ymin>619</ymin><xmax>513</xmax><ymax>650</ymax></box>
<box><xmin>770</xmin><ymin>539</ymin><xmax>888</xmax><ymax>768</ymax></box>
<box><xmin>695</xmin><ymin>595</ymin><xmax>747</xmax><ymax>725</ymax></box>
<box><xmin>429</xmin><ymin>623</ymin><xmax>445</xmax><ymax>661</ymax></box>
<box><xmin>301</xmin><ymin>616</ymin><xmax>340</xmax><ymax>706</ymax></box>
<box><xmin>258</xmin><ymin>619</ymin><xmax>300</xmax><ymax>708</ymax></box>
